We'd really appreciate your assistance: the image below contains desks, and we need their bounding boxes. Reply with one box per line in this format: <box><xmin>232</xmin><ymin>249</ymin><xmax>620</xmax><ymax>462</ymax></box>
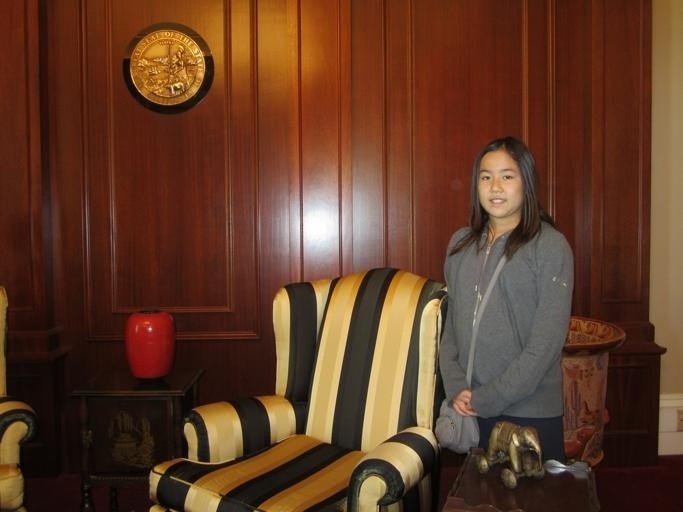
<box><xmin>442</xmin><ymin>444</ymin><xmax>682</xmax><ymax>512</ymax></box>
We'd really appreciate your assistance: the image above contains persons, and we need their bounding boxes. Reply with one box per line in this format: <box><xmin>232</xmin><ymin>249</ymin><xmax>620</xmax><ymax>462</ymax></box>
<box><xmin>433</xmin><ymin>136</ymin><xmax>576</xmax><ymax>468</ymax></box>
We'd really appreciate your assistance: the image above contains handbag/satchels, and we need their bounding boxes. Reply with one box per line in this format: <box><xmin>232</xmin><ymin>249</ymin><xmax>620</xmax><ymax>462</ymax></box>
<box><xmin>435</xmin><ymin>399</ymin><xmax>480</xmax><ymax>454</ymax></box>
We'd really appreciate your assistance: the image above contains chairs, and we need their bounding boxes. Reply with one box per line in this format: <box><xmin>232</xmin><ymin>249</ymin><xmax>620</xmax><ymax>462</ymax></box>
<box><xmin>0</xmin><ymin>285</ymin><xmax>38</xmax><ymax>512</ymax></box>
<box><xmin>143</xmin><ymin>267</ymin><xmax>449</xmax><ymax>512</ymax></box>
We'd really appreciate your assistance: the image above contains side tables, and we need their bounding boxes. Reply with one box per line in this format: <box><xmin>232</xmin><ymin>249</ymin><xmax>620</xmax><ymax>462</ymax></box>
<box><xmin>67</xmin><ymin>363</ymin><xmax>208</xmax><ymax>512</ymax></box>
